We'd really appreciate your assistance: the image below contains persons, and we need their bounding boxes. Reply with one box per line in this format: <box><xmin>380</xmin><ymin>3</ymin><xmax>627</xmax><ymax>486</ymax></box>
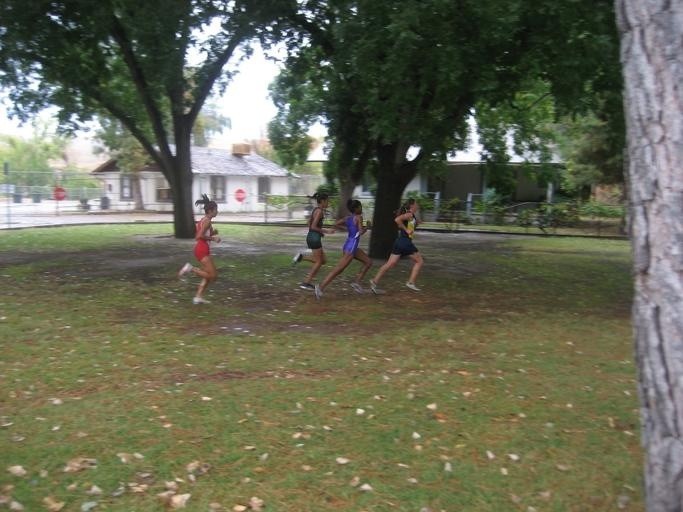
<box><xmin>177</xmin><ymin>193</ymin><xmax>221</xmax><ymax>307</ymax></box>
<box><xmin>313</xmin><ymin>198</ymin><xmax>373</xmax><ymax>300</ymax></box>
<box><xmin>366</xmin><ymin>196</ymin><xmax>424</xmax><ymax>295</ymax></box>
<box><xmin>292</xmin><ymin>191</ymin><xmax>335</xmax><ymax>292</ymax></box>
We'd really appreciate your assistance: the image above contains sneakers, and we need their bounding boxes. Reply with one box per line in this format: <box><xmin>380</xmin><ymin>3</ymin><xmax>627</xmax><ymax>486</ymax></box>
<box><xmin>297</xmin><ymin>282</ymin><xmax>324</xmax><ymax>300</ymax></box>
<box><xmin>193</xmin><ymin>297</ymin><xmax>211</xmax><ymax>304</ymax></box>
<box><xmin>368</xmin><ymin>280</ymin><xmax>377</xmax><ymax>294</ymax></box>
<box><xmin>178</xmin><ymin>262</ymin><xmax>193</xmax><ymax>278</ymax></box>
<box><xmin>350</xmin><ymin>283</ymin><xmax>364</xmax><ymax>295</ymax></box>
<box><xmin>293</xmin><ymin>251</ymin><xmax>303</xmax><ymax>264</ymax></box>
<box><xmin>406</xmin><ymin>282</ymin><xmax>421</xmax><ymax>292</ymax></box>
